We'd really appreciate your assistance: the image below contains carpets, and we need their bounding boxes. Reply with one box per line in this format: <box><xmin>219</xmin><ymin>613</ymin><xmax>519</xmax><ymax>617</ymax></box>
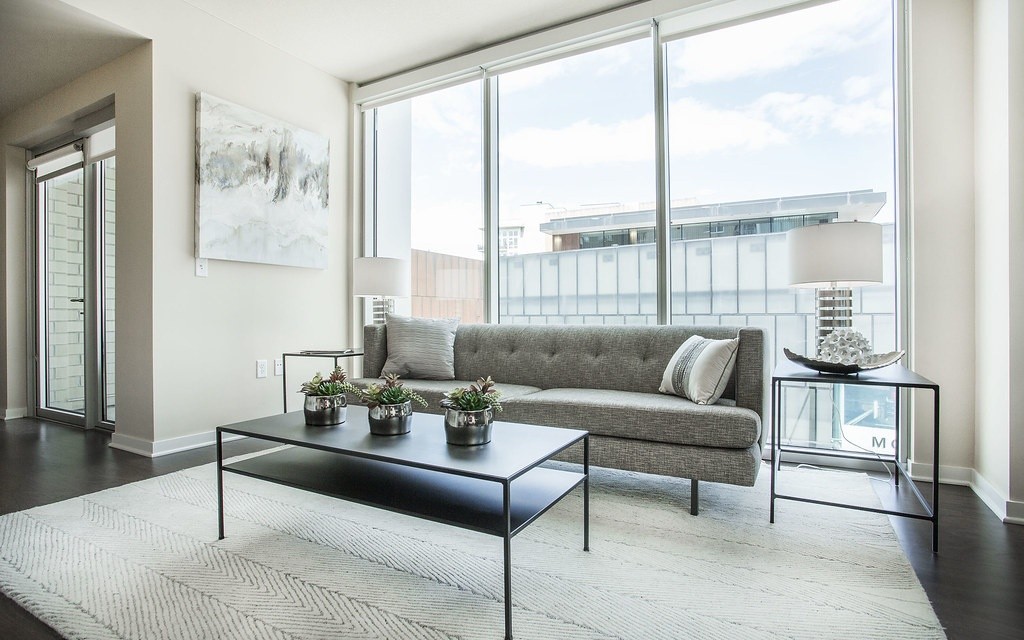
<box><xmin>0</xmin><ymin>445</ymin><xmax>945</xmax><ymax>639</ymax></box>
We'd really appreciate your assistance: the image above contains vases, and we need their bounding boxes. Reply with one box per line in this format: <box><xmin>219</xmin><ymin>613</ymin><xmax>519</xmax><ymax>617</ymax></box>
<box><xmin>304</xmin><ymin>397</ymin><xmax>347</xmax><ymax>425</ymax></box>
<box><xmin>444</xmin><ymin>408</ymin><xmax>494</xmax><ymax>445</ymax></box>
<box><xmin>368</xmin><ymin>401</ymin><xmax>412</xmax><ymax>435</ymax></box>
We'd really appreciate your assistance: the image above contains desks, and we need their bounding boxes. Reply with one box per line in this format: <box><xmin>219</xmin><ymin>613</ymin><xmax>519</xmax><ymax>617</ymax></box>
<box><xmin>216</xmin><ymin>408</ymin><xmax>589</xmax><ymax>640</ymax></box>
<box><xmin>771</xmin><ymin>363</ymin><xmax>940</xmax><ymax>553</ymax></box>
<box><xmin>283</xmin><ymin>351</ymin><xmax>364</xmax><ymax>413</ymax></box>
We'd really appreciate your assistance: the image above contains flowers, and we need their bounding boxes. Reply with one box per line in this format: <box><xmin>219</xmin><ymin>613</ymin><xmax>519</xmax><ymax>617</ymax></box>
<box><xmin>297</xmin><ymin>363</ymin><xmax>363</xmax><ymax>397</ymax></box>
<box><xmin>359</xmin><ymin>372</ymin><xmax>428</xmax><ymax>407</ymax></box>
<box><xmin>441</xmin><ymin>376</ymin><xmax>503</xmax><ymax>413</ymax></box>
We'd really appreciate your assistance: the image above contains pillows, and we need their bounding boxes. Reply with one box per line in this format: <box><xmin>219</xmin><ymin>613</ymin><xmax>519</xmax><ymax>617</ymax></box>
<box><xmin>658</xmin><ymin>334</ymin><xmax>741</xmax><ymax>407</ymax></box>
<box><xmin>379</xmin><ymin>313</ymin><xmax>460</xmax><ymax>381</ymax></box>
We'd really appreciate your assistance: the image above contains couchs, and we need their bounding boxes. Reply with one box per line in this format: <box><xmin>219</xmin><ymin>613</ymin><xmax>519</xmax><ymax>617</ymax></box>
<box><xmin>342</xmin><ymin>323</ymin><xmax>768</xmax><ymax>512</ymax></box>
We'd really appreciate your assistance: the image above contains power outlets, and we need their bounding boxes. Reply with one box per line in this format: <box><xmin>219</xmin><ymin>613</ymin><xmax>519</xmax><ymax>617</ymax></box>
<box><xmin>256</xmin><ymin>360</ymin><xmax>267</xmax><ymax>378</ymax></box>
<box><xmin>274</xmin><ymin>360</ymin><xmax>284</xmax><ymax>376</ymax></box>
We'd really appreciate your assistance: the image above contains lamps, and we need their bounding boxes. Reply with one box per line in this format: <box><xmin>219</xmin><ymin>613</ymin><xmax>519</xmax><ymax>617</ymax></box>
<box><xmin>784</xmin><ymin>221</ymin><xmax>905</xmax><ymax>331</ymax></box>
<box><xmin>353</xmin><ymin>257</ymin><xmax>412</xmax><ymax>322</ymax></box>
